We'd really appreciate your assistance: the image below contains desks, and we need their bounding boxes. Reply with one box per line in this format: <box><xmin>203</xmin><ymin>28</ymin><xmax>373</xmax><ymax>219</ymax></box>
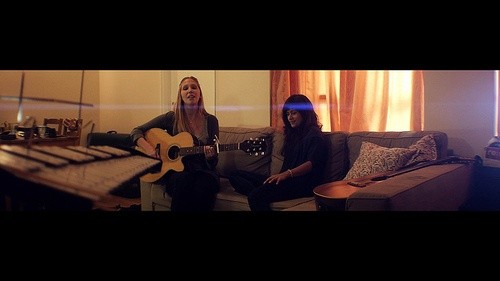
<box><xmin>0</xmin><ymin>134</ymin><xmax>80</xmax><ymax>146</ymax></box>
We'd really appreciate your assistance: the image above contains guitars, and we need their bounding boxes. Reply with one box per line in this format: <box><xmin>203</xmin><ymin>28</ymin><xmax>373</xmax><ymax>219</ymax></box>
<box><xmin>135</xmin><ymin>128</ymin><xmax>268</xmax><ymax>184</ymax></box>
<box><xmin>312</xmin><ymin>155</ymin><xmax>460</xmax><ymax>213</ymax></box>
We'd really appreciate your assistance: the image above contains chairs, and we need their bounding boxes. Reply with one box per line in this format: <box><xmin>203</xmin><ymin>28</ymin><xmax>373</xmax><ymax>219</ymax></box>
<box><xmin>64</xmin><ymin>118</ymin><xmax>84</xmax><ymax>146</ymax></box>
<box><xmin>43</xmin><ymin>117</ymin><xmax>62</xmax><ymax>135</ymax></box>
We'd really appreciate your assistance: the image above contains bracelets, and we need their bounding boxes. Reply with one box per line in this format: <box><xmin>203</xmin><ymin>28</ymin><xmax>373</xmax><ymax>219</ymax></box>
<box><xmin>287</xmin><ymin>169</ymin><xmax>293</xmax><ymax>176</ymax></box>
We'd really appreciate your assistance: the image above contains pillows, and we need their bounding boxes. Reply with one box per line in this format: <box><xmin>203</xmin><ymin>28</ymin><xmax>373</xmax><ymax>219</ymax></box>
<box><xmin>407</xmin><ymin>132</ymin><xmax>438</xmax><ymax>165</ymax></box>
<box><xmin>345</xmin><ymin>140</ymin><xmax>420</xmax><ymax>186</ymax></box>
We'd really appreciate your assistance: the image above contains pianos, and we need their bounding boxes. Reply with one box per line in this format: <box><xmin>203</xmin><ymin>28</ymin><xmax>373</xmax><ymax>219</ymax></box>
<box><xmin>0</xmin><ymin>145</ymin><xmax>164</xmax><ymax>209</ymax></box>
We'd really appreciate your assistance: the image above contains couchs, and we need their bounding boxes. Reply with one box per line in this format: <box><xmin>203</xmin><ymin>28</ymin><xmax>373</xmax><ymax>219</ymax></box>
<box><xmin>140</xmin><ymin>127</ymin><xmax>477</xmax><ymax>209</ymax></box>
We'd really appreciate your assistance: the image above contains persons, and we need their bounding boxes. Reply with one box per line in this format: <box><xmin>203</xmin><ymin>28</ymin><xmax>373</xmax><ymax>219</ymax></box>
<box><xmin>130</xmin><ymin>76</ymin><xmax>219</xmax><ymax>210</ymax></box>
<box><xmin>229</xmin><ymin>93</ymin><xmax>327</xmax><ymax>210</ymax></box>
